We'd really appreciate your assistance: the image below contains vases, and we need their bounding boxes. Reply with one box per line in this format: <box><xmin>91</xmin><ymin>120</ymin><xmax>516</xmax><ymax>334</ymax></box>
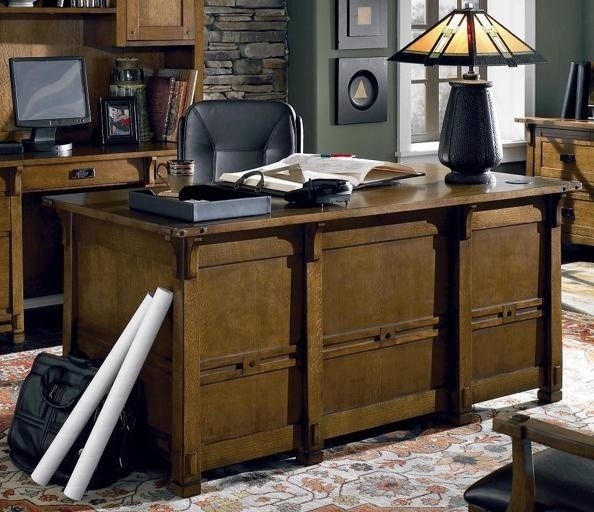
<box><xmin>110</xmin><ymin>56</ymin><xmax>152</xmax><ymax>141</ymax></box>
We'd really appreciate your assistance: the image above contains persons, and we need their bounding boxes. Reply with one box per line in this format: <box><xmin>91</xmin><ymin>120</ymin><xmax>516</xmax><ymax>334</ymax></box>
<box><xmin>110</xmin><ymin>107</ymin><xmax>130</xmax><ymax>131</ymax></box>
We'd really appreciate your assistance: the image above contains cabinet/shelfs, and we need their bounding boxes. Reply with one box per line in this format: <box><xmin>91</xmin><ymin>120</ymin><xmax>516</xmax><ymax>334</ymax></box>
<box><xmin>1</xmin><ymin>1</ymin><xmax>204</xmax><ymax>152</ymax></box>
<box><xmin>515</xmin><ymin>113</ymin><xmax>593</xmax><ymax>246</ymax></box>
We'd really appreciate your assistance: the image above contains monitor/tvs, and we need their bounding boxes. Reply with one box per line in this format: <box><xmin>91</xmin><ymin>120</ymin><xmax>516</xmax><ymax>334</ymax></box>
<box><xmin>8</xmin><ymin>55</ymin><xmax>92</xmax><ymax>151</ymax></box>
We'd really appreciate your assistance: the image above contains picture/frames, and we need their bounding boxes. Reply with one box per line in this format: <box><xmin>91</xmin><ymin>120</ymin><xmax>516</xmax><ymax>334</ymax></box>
<box><xmin>98</xmin><ymin>95</ymin><xmax>139</xmax><ymax>145</ymax></box>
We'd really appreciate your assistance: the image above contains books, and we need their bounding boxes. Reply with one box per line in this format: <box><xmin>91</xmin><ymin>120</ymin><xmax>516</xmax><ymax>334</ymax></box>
<box><xmin>219</xmin><ymin>154</ymin><xmax>425</xmax><ymax>193</ymax></box>
<box><xmin>151</xmin><ymin>68</ymin><xmax>199</xmax><ymax>143</ymax></box>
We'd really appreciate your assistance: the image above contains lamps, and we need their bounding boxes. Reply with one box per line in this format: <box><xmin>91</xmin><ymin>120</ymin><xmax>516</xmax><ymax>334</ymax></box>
<box><xmin>385</xmin><ymin>2</ymin><xmax>547</xmax><ymax>184</ymax></box>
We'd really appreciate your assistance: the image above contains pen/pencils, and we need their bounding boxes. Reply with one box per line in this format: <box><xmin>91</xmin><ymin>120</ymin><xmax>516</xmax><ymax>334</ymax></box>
<box><xmin>320</xmin><ymin>153</ymin><xmax>353</xmax><ymax>157</ymax></box>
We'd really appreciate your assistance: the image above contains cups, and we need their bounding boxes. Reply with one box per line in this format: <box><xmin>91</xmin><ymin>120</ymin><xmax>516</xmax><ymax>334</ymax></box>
<box><xmin>157</xmin><ymin>159</ymin><xmax>195</xmax><ymax>193</ymax></box>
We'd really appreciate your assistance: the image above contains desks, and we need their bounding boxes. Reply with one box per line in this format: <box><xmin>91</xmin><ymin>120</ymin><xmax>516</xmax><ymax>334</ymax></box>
<box><xmin>0</xmin><ymin>148</ymin><xmax>181</xmax><ymax>344</ymax></box>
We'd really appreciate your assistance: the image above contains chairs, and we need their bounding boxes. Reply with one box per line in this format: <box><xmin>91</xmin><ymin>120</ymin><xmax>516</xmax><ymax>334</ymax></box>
<box><xmin>174</xmin><ymin>98</ymin><xmax>304</xmax><ymax>186</ymax></box>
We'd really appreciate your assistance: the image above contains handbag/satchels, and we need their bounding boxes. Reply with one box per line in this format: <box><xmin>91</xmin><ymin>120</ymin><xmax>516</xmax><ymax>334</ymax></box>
<box><xmin>7</xmin><ymin>353</ymin><xmax>147</xmax><ymax>490</ymax></box>
